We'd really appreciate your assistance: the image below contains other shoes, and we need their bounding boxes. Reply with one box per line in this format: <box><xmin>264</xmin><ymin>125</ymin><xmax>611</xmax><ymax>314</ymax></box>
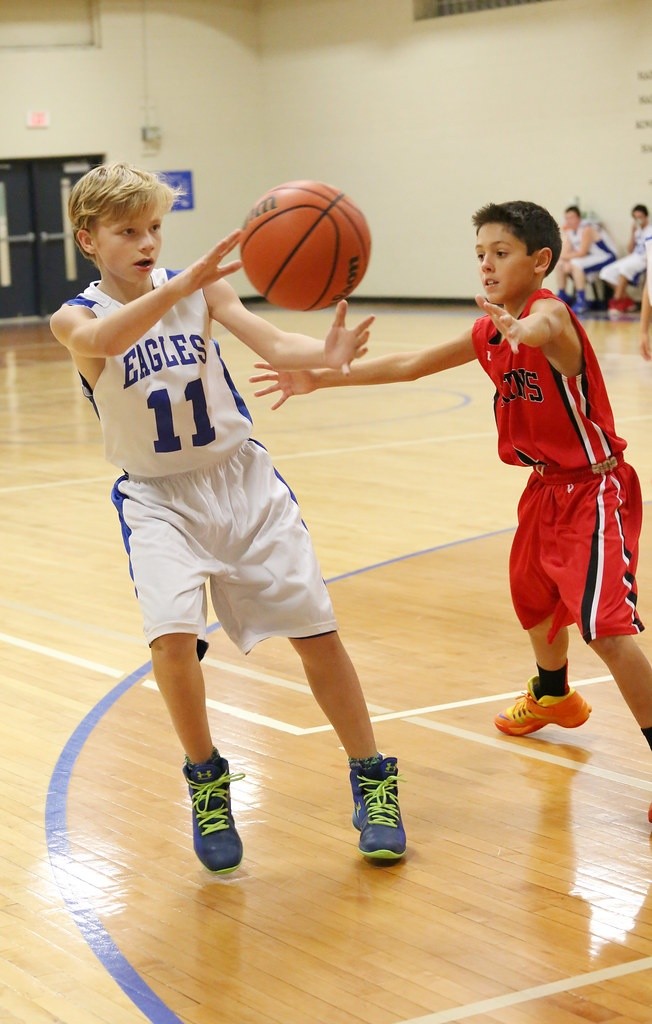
<box><xmin>622</xmin><ymin>296</ymin><xmax>637</xmax><ymax>313</ymax></box>
<box><xmin>572</xmin><ymin>298</ymin><xmax>592</xmax><ymax>314</ymax></box>
<box><xmin>556</xmin><ymin>295</ymin><xmax>568</xmax><ymax>306</ymax></box>
<box><xmin>607</xmin><ymin>297</ymin><xmax>624</xmax><ymax>318</ymax></box>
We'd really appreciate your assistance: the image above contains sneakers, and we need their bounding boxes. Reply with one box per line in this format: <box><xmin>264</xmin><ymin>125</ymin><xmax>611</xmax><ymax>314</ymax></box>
<box><xmin>178</xmin><ymin>755</ymin><xmax>248</xmax><ymax>878</ymax></box>
<box><xmin>494</xmin><ymin>673</ymin><xmax>596</xmax><ymax>737</ymax></box>
<box><xmin>346</xmin><ymin>757</ymin><xmax>407</xmax><ymax>861</ymax></box>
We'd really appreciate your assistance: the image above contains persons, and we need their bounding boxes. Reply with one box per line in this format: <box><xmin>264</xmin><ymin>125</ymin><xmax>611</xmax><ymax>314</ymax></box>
<box><xmin>553</xmin><ymin>205</ymin><xmax>615</xmax><ymax>316</ymax></box>
<box><xmin>50</xmin><ymin>160</ymin><xmax>411</xmax><ymax>873</ymax></box>
<box><xmin>599</xmin><ymin>204</ymin><xmax>650</xmax><ymax>320</ymax></box>
<box><xmin>638</xmin><ymin>229</ymin><xmax>652</xmax><ymax>361</ymax></box>
<box><xmin>252</xmin><ymin>200</ymin><xmax>652</xmax><ymax>834</ymax></box>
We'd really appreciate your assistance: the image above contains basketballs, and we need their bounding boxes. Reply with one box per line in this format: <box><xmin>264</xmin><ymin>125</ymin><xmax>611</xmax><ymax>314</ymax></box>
<box><xmin>240</xmin><ymin>183</ymin><xmax>373</xmax><ymax>312</ymax></box>
